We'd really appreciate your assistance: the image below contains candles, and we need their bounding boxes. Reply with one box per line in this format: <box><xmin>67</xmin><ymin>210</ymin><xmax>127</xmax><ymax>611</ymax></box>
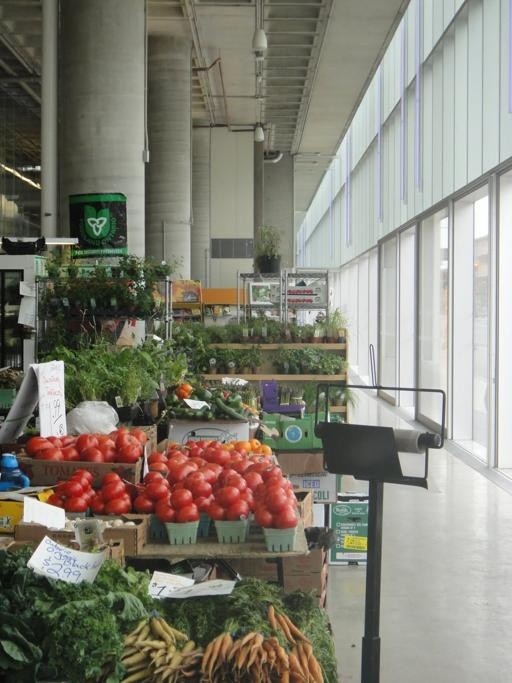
<box><xmin>62</xmin><ymin>335</ymin><xmax>186</xmax><ymax>419</ymax></box>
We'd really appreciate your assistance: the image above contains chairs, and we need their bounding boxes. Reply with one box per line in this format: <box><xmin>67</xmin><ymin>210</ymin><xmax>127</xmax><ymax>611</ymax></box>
<box><xmin>260</xmin><ymin>378</ymin><xmax>305</xmax><ymax>419</ymax></box>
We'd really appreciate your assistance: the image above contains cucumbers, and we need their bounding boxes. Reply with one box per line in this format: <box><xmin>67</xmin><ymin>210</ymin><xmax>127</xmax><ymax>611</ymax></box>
<box><xmin>168</xmin><ymin>386</ymin><xmax>250</xmax><ymax>422</ymax></box>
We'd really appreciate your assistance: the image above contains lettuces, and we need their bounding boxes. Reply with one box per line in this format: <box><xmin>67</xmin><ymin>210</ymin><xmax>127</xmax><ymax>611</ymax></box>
<box><xmin>44</xmin><ymin>564</ymin><xmax>140</xmax><ymax>683</ymax></box>
<box><xmin>40</xmin><ymin>345</ymin><xmax>184</xmax><ymax>410</ymax></box>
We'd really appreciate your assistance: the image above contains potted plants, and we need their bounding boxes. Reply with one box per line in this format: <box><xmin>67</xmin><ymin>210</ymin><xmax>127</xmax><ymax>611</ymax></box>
<box><xmin>254</xmin><ymin>223</ymin><xmax>281</xmax><ymax>273</ymax></box>
<box><xmin>175</xmin><ymin>315</ymin><xmax>342</xmax><ymax>374</ymax></box>
<box><xmin>39</xmin><ymin>255</ymin><xmax>178</xmax><ymax>353</ymax></box>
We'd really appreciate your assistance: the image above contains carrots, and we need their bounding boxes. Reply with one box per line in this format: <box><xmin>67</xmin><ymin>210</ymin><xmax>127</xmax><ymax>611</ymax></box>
<box><xmin>85</xmin><ymin>596</ymin><xmax>204</xmax><ymax>683</ymax></box>
<box><xmin>197</xmin><ymin>596</ymin><xmax>334</xmax><ymax>683</ymax></box>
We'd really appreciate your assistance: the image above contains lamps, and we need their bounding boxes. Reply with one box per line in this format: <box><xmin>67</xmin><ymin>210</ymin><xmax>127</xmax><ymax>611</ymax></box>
<box><xmin>252</xmin><ymin>29</ymin><xmax>268</xmax><ymax>51</ymax></box>
<box><xmin>254</xmin><ymin>122</ymin><xmax>264</xmax><ymax>142</ymax></box>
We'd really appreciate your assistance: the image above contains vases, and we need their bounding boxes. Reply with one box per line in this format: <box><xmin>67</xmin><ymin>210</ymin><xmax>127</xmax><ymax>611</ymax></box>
<box><xmin>164</xmin><ymin>519</ymin><xmax>200</xmax><ymax>545</ymax></box>
<box><xmin>196</xmin><ymin>511</ymin><xmax>211</xmax><ymax>537</ymax></box>
<box><xmin>151</xmin><ymin>514</ymin><xmax>166</xmax><ymax>538</ymax></box>
<box><xmin>262</xmin><ymin>526</ymin><xmax>297</xmax><ymax>552</ymax></box>
<box><xmin>215</xmin><ymin>519</ymin><xmax>249</xmax><ymax>544</ymax></box>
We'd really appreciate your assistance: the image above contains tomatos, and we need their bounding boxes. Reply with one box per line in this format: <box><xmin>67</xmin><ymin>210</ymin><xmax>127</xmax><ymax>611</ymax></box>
<box><xmin>42</xmin><ymin>462</ymin><xmax>133</xmax><ymax>524</ymax></box>
<box><xmin>137</xmin><ymin>438</ymin><xmax>301</xmax><ymax>535</ymax></box>
<box><xmin>27</xmin><ymin>428</ymin><xmax>147</xmax><ymax>466</ymax></box>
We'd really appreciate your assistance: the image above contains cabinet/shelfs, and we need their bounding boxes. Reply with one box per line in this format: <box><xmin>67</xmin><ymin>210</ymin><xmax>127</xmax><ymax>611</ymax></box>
<box><xmin>285</xmin><ymin>267</ymin><xmax>329</xmax><ymax>326</ymax></box>
<box><xmin>237</xmin><ymin>268</ymin><xmax>283</xmax><ymax>323</ymax></box>
<box><xmin>0</xmin><ymin>255</ymin><xmax>36</xmax><ymax>373</ymax></box>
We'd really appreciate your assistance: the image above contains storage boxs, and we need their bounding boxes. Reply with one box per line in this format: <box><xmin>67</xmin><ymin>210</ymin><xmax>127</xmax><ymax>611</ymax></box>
<box><xmin>265</xmin><ymin>564</ymin><xmax>329</xmax><ymax>598</ymax></box>
<box><xmin>328</xmin><ymin>491</ymin><xmax>371</xmax><ymax>562</ymax></box>
<box><xmin>145</xmin><ymin>424</ymin><xmax>160</xmax><ymax>459</ymax></box>
<box><xmin>7</xmin><ymin>538</ymin><xmax>125</xmax><ymax>563</ymax></box>
<box><xmin>277</xmin><ymin>453</ymin><xmax>338</xmax><ymax>503</ymax></box>
<box><xmin>27</xmin><ymin>458</ymin><xmax>143</xmax><ymax>488</ymax></box>
<box><xmin>312</xmin><ymin>591</ymin><xmax>330</xmax><ymax>609</ymax></box>
<box><xmin>14</xmin><ymin>516</ymin><xmax>148</xmax><ymax>555</ymax></box>
<box><xmin>261</xmin><ymin>419</ymin><xmax>326</xmax><ymax>451</ymax></box>
<box><xmin>168</xmin><ymin>418</ymin><xmax>250</xmax><ymax>445</ymax></box>
<box><xmin>251</xmin><ymin>544</ymin><xmax>331</xmax><ymax>575</ymax></box>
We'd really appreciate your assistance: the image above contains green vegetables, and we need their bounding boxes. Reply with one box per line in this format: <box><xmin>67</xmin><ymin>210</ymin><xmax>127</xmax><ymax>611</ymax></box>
<box><xmin>1</xmin><ymin>552</ymin><xmax>44</xmax><ymax>679</ymax></box>
